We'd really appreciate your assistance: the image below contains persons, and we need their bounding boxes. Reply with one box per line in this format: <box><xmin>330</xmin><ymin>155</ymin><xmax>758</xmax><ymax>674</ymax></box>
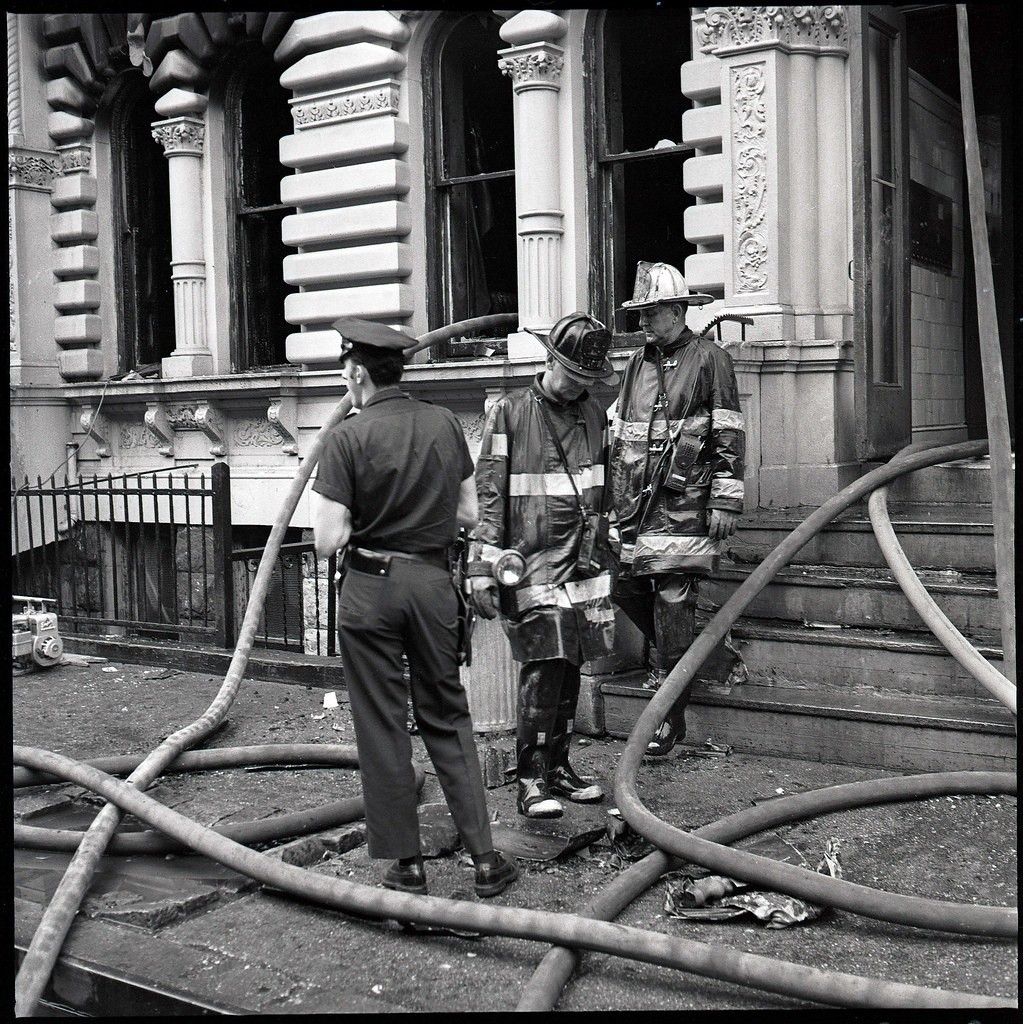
<box><xmin>311</xmin><ymin>317</ymin><xmax>520</xmax><ymax>897</ymax></box>
<box><xmin>606</xmin><ymin>263</ymin><xmax>746</xmax><ymax>765</ymax></box>
<box><xmin>470</xmin><ymin>313</ymin><xmax>619</xmax><ymax>815</ymax></box>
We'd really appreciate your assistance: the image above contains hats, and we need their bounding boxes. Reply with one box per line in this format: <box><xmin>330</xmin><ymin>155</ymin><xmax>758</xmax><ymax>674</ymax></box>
<box><xmin>331</xmin><ymin>317</ymin><xmax>418</xmax><ymax>362</ymax></box>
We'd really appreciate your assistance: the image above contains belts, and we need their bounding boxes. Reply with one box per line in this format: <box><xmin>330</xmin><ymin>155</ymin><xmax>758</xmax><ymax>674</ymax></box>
<box><xmin>351</xmin><ymin>540</ymin><xmax>450</xmax><ymax>570</ymax></box>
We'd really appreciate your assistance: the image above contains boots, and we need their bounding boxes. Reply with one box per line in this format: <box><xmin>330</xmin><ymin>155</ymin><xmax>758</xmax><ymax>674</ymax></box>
<box><xmin>553</xmin><ymin>719</ymin><xmax>603</xmax><ymax>802</ymax></box>
<box><xmin>516</xmin><ymin>732</ymin><xmax>564</xmax><ymax>817</ymax></box>
<box><xmin>644</xmin><ymin>669</ymin><xmax>692</xmax><ymax>756</ymax></box>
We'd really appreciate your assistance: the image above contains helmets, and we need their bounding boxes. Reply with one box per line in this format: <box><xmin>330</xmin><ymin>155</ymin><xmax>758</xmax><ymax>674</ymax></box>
<box><xmin>621</xmin><ymin>261</ymin><xmax>715</xmax><ymax>306</ymax></box>
<box><xmin>524</xmin><ymin>311</ymin><xmax>615</xmax><ymax>378</ymax></box>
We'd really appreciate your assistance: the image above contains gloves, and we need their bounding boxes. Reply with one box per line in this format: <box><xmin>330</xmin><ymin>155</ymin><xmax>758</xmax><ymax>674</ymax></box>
<box><xmin>469</xmin><ymin>576</ymin><xmax>499</xmax><ymax>620</ymax></box>
<box><xmin>706</xmin><ymin>508</ymin><xmax>738</xmax><ymax>541</ymax></box>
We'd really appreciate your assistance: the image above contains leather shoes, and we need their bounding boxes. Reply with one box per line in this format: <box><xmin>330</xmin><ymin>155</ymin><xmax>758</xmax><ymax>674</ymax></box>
<box><xmin>381</xmin><ymin>860</ymin><xmax>428</xmax><ymax>895</ymax></box>
<box><xmin>473</xmin><ymin>852</ymin><xmax>520</xmax><ymax>898</ymax></box>
